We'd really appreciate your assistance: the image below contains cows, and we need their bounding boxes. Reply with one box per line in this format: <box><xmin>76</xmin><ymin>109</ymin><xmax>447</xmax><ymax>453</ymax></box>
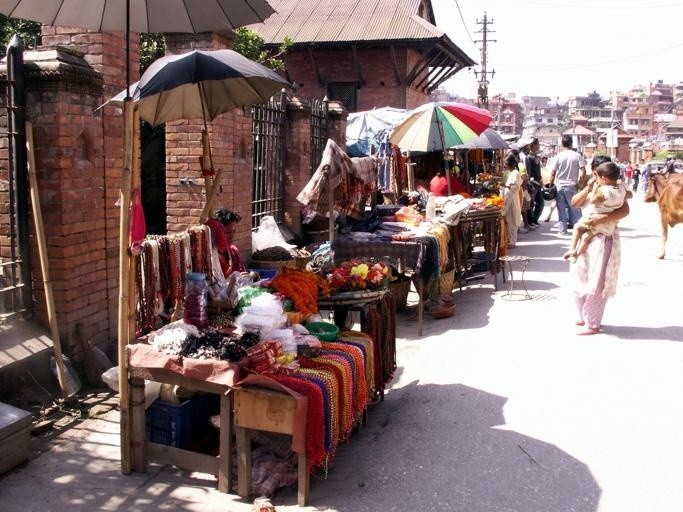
<box><xmin>644</xmin><ymin>171</ymin><xmax>683</xmax><ymax>260</ymax></box>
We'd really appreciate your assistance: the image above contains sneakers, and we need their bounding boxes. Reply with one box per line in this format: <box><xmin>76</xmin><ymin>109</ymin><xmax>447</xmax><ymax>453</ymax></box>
<box><xmin>518</xmin><ymin>222</ymin><xmax>540</xmax><ymax>233</ymax></box>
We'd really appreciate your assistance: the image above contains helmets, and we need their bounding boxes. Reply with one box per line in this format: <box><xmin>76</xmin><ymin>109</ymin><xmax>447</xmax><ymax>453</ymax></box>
<box><xmin>542</xmin><ymin>183</ymin><xmax>557</xmax><ymax>200</ymax></box>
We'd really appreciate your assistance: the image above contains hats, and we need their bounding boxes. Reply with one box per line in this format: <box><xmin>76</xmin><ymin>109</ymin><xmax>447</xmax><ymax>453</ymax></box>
<box><xmin>518</xmin><ymin>137</ymin><xmax>534</xmax><ymax>148</ymax></box>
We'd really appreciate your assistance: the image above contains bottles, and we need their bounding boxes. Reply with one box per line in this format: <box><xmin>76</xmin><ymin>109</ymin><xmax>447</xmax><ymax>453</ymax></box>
<box><xmin>183</xmin><ymin>273</ymin><xmax>211</xmax><ymax>327</ymax></box>
<box><xmin>425</xmin><ymin>192</ymin><xmax>436</xmax><ymax>222</ymax></box>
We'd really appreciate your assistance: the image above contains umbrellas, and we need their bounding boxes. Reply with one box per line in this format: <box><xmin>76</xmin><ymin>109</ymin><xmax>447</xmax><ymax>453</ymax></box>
<box><xmin>449</xmin><ymin>128</ymin><xmax>509</xmax><ymax>182</ymax></box>
<box><xmin>388</xmin><ymin>101</ymin><xmax>494</xmax><ymax>196</ymax></box>
<box><xmin>94</xmin><ymin>81</ymin><xmax>140</xmax><ymax>112</ymax></box>
<box><xmin>132</xmin><ymin>49</ymin><xmax>292</xmax><ymax>196</ymax></box>
<box><xmin>0</xmin><ymin>0</ymin><xmax>278</xmax><ymax>97</ymax></box>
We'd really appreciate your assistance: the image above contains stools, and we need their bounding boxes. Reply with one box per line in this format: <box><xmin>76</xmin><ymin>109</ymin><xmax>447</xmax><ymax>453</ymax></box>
<box><xmin>498</xmin><ymin>256</ymin><xmax>533</xmax><ymax>301</ymax></box>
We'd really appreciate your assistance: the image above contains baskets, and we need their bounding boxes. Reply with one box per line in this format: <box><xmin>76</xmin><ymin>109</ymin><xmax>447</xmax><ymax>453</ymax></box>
<box><xmin>146</xmin><ymin>398</ymin><xmax>192</xmax><ymax>449</ymax></box>
<box><xmin>413</xmin><ymin>267</ymin><xmax>456</xmax><ymax>301</ymax></box>
<box><xmin>429</xmin><ymin>304</ymin><xmax>456</xmax><ymax>319</ymax></box>
<box><xmin>391</xmin><ymin>277</ymin><xmax>411</xmax><ymax>313</ymax></box>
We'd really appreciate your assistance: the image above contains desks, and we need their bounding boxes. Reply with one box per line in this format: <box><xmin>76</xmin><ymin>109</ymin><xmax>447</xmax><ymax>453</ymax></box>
<box><xmin>127</xmin><ymin>343</ymin><xmax>236</xmax><ymax>492</ymax></box>
<box><xmin>230</xmin><ymin>386</ymin><xmax>310</xmax><ymax>506</ymax></box>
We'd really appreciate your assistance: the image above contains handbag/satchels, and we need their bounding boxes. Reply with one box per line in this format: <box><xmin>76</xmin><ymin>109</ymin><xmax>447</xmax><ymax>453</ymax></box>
<box><xmin>230</xmin><ymin>430</ymin><xmax>299</xmax><ymax>500</ymax></box>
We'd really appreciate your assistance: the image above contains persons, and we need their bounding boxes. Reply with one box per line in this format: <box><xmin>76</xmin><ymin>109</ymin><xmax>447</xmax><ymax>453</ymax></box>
<box><xmin>215</xmin><ymin>208</ymin><xmax>244</xmax><ymax>279</ymax></box>
<box><xmin>499</xmin><ymin>133</ymin><xmax>654</xmax><ymax>335</ymax></box>
<box><xmin>429</xmin><ymin>160</ymin><xmax>466</xmax><ymax>196</ymax></box>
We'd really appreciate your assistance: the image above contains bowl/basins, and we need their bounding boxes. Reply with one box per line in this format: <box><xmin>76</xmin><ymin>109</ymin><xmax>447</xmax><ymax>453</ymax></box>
<box><xmin>305</xmin><ymin>322</ymin><xmax>339</xmax><ymax>340</ymax></box>
